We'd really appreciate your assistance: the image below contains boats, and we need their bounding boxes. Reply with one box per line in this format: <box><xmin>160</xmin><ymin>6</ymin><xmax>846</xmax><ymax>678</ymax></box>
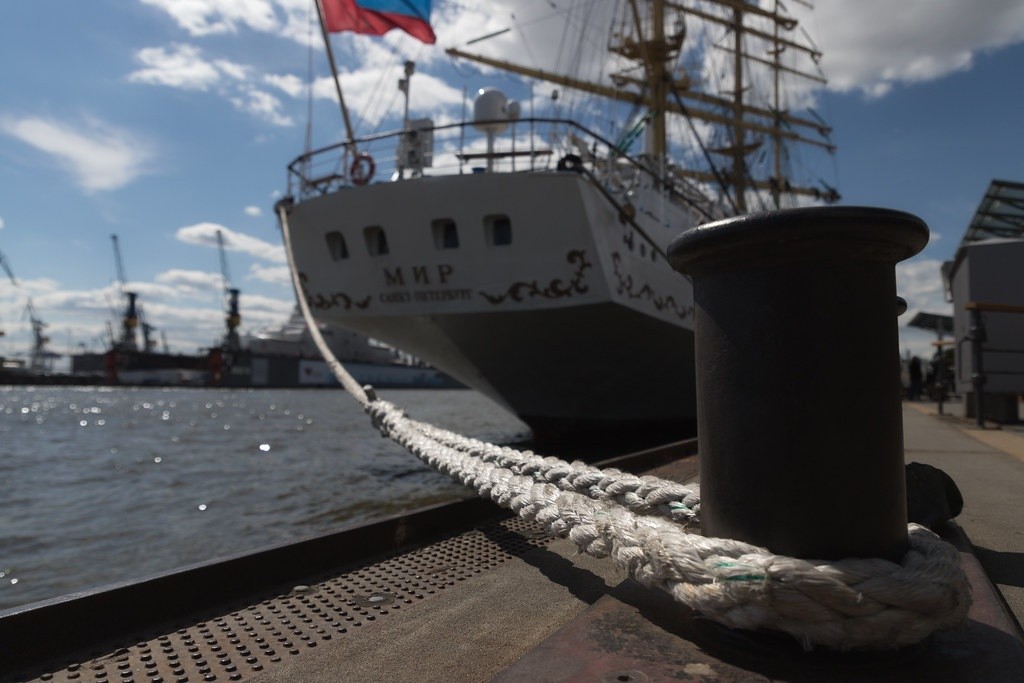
<box><xmin>1</xmin><ymin>229</ymin><xmax>470</xmax><ymax>394</ymax></box>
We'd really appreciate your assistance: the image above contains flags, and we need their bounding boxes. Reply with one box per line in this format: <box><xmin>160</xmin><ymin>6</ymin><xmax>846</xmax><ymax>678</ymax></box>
<box><xmin>316</xmin><ymin>0</ymin><xmax>438</xmax><ymax>45</ymax></box>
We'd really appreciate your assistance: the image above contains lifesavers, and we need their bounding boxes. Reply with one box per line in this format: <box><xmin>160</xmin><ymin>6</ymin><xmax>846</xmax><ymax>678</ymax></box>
<box><xmin>350</xmin><ymin>154</ymin><xmax>375</xmax><ymax>186</ymax></box>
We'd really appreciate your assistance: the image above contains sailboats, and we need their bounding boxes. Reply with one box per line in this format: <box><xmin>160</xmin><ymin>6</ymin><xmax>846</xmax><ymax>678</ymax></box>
<box><xmin>273</xmin><ymin>0</ymin><xmax>845</xmax><ymax>456</ymax></box>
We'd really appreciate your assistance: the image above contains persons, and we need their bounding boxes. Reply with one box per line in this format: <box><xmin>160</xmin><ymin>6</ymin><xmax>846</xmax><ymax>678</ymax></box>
<box><xmin>906</xmin><ymin>356</ymin><xmax>924</xmax><ymax>402</ymax></box>
